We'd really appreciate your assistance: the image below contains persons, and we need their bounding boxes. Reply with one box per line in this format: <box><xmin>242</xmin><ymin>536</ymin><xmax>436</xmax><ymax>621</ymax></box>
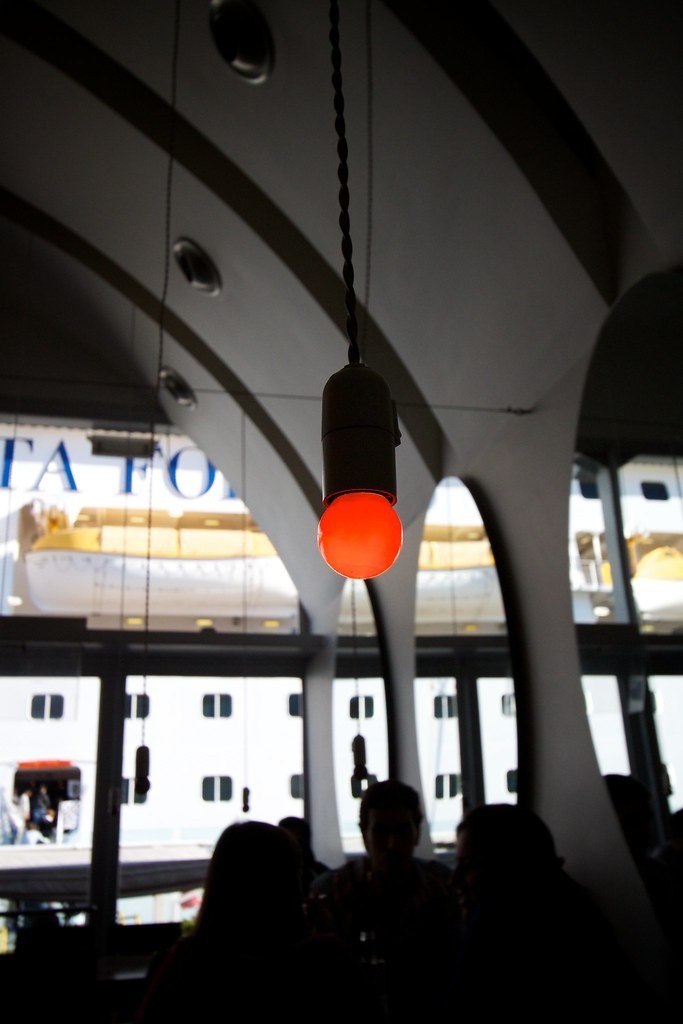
<box><xmin>299</xmin><ymin>781</ymin><xmax>466</xmax><ymax>1023</ymax></box>
<box><xmin>605</xmin><ymin>775</ymin><xmax>682</xmax><ymax>961</ymax></box>
<box><xmin>278</xmin><ymin>818</ymin><xmax>332</xmax><ymax>887</ymax></box>
<box><xmin>437</xmin><ymin>804</ymin><xmax>675</xmax><ymax>1024</ymax></box>
<box><xmin>132</xmin><ymin>823</ymin><xmax>391</xmax><ymax>1024</ymax></box>
<box><xmin>16</xmin><ymin>786</ymin><xmax>52</xmax><ymax>845</ymax></box>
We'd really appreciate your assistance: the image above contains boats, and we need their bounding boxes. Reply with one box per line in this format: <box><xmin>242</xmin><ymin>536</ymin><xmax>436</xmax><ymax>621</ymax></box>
<box><xmin>19</xmin><ymin>498</ymin><xmax>506</xmax><ymax>632</ymax></box>
<box><xmin>0</xmin><ymin>412</ymin><xmax>682</xmax><ymax>925</ymax></box>
<box><xmin>625</xmin><ymin>542</ymin><xmax>683</xmax><ymax>634</ymax></box>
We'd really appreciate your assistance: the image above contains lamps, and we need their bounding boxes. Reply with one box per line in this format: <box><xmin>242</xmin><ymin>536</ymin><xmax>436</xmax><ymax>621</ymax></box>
<box><xmin>312</xmin><ymin>1</ymin><xmax>404</xmax><ymax>581</ymax></box>
